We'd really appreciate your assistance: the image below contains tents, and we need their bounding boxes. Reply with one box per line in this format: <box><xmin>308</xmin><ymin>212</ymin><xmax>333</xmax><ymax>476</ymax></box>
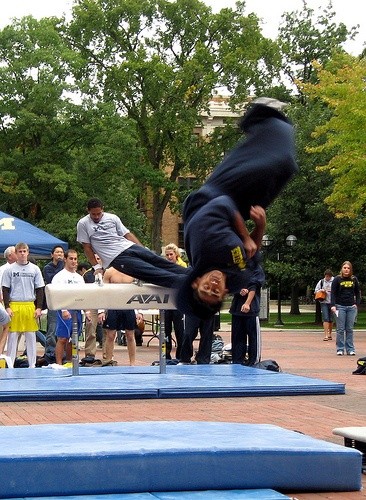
<box><xmin>0</xmin><ymin>209</ymin><xmax>69</xmax><ymax>256</ymax></box>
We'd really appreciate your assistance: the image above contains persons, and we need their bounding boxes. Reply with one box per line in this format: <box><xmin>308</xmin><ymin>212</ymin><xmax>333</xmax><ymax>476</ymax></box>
<box><xmin>98</xmin><ymin>264</ymin><xmax>137</xmax><ymax>366</ymax></box>
<box><xmin>76</xmin><ymin>198</ymin><xmax>193</xmax><ymax>289</ymax></box>
<box><xmin>331</xmin><ymin>261</ymin><xmax>360</xmax><ymax>355</ymax></box>
<box><xmin>51</xmin><ymin>250</ymin><xmax>86</xmax><ymax>365</ymax></box>
<box><xmin>177</xmin><ymin>98</ymin><xmax>297</xmax><ymax>319</ymax></box>
<box><xmin>177</xmin><ymin>315</ymin><xmax>214</xmax><ymax>366</ymax></box>
<box><xmin>1</xmin><ymin>241</ymin><xmax>45</xmax><ymax>368</ymax></box>
<box><xmin>77</xmin><ymin>263</ymin><xmax>104</xmax><ymax>348</ymax></box>
<box><xmin>228</xmin><ymin>250</ymin><xmax>265</xmax><ymax>365</ymax></box>
<box><xmin>0</xmin><ymin>246</ymin><xmax>21</xmax><ymax>356</ymax></box>
<box><xmin>0</xmin><ymin>302</ymin><xmax>12</xmax><ymax>356</ymax></box>
<box><xmin>162</xmin><ymin>243</ymin><xmax>187</xmax><ymax>364</ymax></box>
<box><xmin>81</xmin><ymin>252</ymin><xmax>110</xmax><ymax>362</ymax></box>
<box><xmin>43</xmin><ymin>246</ymin><xmax>66</xmax><ymax>365</ymax></box>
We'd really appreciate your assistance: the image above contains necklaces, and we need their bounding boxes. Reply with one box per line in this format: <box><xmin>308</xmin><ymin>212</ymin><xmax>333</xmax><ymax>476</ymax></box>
<box><xmin>314</xmin><ymin>269</ymin><xmax>335</xmax><ymax>341</ymax></box>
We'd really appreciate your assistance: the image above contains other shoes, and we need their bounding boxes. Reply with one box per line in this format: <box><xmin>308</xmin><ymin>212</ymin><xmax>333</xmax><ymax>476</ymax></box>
<box><xmin>349</xmin><ymin>350</ymin><xmax>356</xmax><ymax>355</ymax></box>
<box><xmin>337</xmin><ymin>350</ymin><xmax>344</xmax><ymax>355</ymax></box>
<box><xmin>324</xmin><ymin>336</ymin><xmax>328</xmax><ymax>341</ymax></box>
<box><xmin>328</xmin><ymin>336</ymin><xmax>332</xmax><ymax>340</ymax></box>
